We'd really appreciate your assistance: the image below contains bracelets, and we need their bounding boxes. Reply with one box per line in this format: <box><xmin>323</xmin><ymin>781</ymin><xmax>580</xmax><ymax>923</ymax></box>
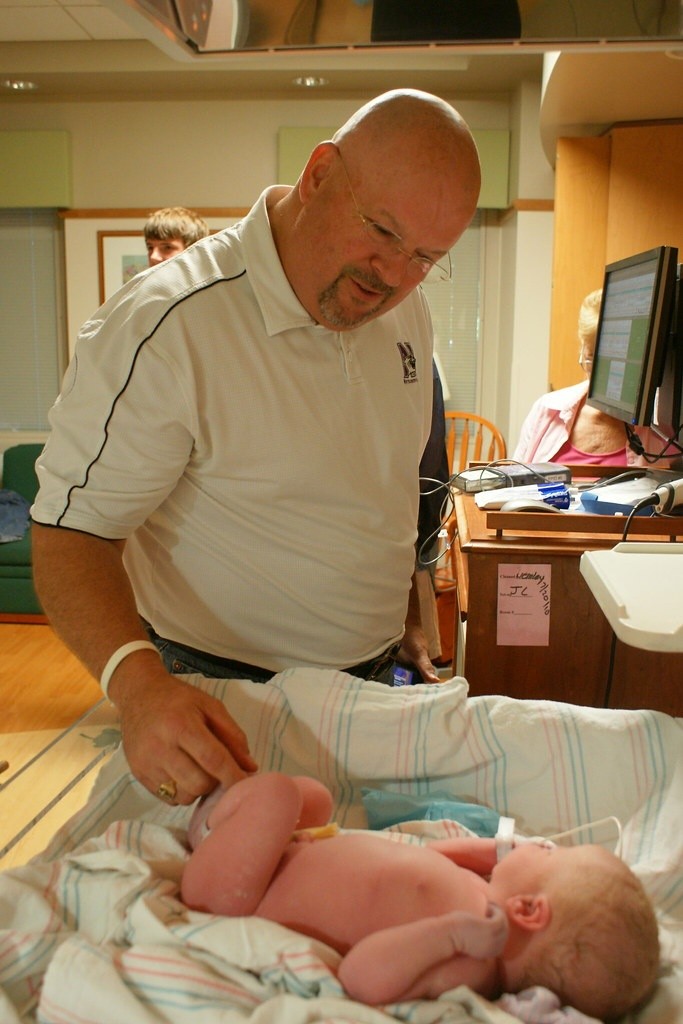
<box><xmin>100</xmin><ymin>640</ymin><xmax>164</xmax><ymax>708</ymax></box>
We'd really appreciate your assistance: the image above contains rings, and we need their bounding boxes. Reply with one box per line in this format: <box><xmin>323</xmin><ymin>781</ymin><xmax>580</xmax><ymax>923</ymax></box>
<box><xmin>157</xmin><ymin>779</ymin><xmax>176</xmax><ymax>801</ymax></box>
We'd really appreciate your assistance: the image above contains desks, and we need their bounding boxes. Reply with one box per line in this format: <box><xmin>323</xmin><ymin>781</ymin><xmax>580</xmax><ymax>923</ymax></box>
<box><xmin>430</xmin><ymin>410</ymin><xmax>504</xmax><ymax>667</ymax></box>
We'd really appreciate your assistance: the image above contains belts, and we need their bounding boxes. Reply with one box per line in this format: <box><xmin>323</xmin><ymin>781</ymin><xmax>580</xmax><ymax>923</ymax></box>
<box><xmin>147</xmin><ymin>627</ymin><xmax>402</xmax><ymax>682</ymax></box>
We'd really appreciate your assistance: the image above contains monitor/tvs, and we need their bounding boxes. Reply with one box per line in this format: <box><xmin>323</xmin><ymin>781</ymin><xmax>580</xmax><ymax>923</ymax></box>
<box><xmin>586</xmin><ymin>245</ymin><xmax>683</xmax><ymax>441</ymax></box>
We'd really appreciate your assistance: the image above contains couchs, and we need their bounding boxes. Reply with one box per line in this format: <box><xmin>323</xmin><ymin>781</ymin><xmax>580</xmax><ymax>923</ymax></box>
<box><xmin>0</xmin><ymin>444</ymin><xmax>48</xmax><ymax>614</ymax></box>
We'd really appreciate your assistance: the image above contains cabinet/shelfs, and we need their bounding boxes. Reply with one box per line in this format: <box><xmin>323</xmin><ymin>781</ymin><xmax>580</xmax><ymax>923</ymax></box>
<box><xmin>453</xmin><ymin>487</ymin><xmax>681</xmax><ymax>722</ymax></box>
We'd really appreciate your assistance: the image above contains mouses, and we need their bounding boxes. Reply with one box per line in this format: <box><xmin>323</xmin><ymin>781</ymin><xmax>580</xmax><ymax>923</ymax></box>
<box><xmin>500</xmin><ymin>498</ymin><xmax>564</xmax><ymax>514</ymax></box>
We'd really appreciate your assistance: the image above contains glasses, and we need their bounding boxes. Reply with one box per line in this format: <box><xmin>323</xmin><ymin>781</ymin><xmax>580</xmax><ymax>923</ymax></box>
<box><xmin>579</xmin><ymin>343</ymin><xmax>593</xmax><ymax>374</ymax></box>
<box><xmin>336</xmin><ymin>148</ymin><xmax>451</xmax><ymax>284</ymax></box>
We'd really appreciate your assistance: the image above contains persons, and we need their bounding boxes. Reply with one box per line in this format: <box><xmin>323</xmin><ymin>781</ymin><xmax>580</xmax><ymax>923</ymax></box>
<box><xmin>181</xmin><ymin>773</ymin><xmax>660</xmax><ymax>1020</ymax></box>
<box><xmin>511</xmin><ymin>289</ymin><xmax>683</xmax><ymax>471</ymax></box>
<box><xmin>30</xmin><ymin>89</ymin><xmax>480</xmax><ymax>807</ymax></box>
